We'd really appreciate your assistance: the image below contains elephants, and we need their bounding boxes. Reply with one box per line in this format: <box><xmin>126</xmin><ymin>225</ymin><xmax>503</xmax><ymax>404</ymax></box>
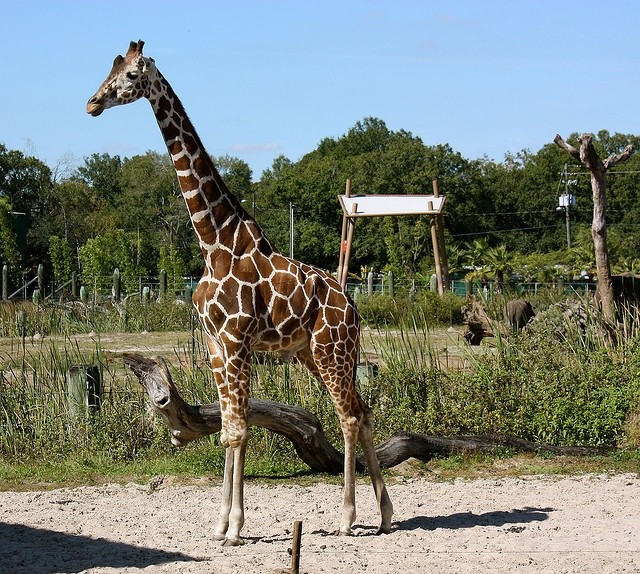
<box><xmin>594</xmin><ymin>273</ymin><xmax>640</xmax><ymax>318</ymax></box>
<box><xmin>505</xmin><ymin>299</ymin><xmax>536</xmax><ymax>331</ymax></box>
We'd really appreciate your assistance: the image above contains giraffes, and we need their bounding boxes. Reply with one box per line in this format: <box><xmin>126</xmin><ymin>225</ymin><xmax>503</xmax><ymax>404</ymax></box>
<box><xmin>86</xmin><ymin>40</ymin><xmax>392</xmax><ymax>547</ymax></box>
